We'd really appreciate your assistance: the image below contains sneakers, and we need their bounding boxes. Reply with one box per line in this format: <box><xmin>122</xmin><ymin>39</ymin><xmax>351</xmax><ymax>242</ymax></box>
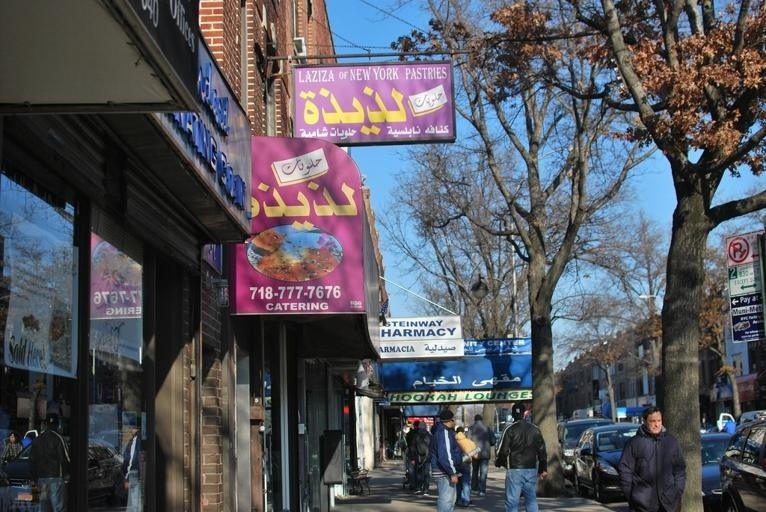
<box><xmin>471</xmin><ymin>490</ymin><xmax>485</xmax><ymax>497</ymax></box>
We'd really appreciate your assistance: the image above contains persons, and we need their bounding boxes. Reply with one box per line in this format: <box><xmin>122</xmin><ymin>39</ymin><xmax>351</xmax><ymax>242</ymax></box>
<box><xmin>617</xmin><ymin>406</ymin><xmax>687</xmax><ymax>511</ymax></box>
<box><xmin>0</xmin><ymin>430</ymin><xmax>23</xmax><ymax>459</ymax></box>
<box><xmin>30</xmin><ymin>413</ymin><xmax>71</xmax><ymax>511</ymax></box>
<box><xmin>399</xmin><ymin>410</ymin><xmax>497</xmax><ymax>511</ymax></box>
<box><xmin>494</xmin><ymin>402</ymin><xmax>547</xmax><ymax>511</ymax></box>
<box><xmin>120</xmin><ymin>416</ymin><xmax>144</xmax><ymax>511</ymax></box>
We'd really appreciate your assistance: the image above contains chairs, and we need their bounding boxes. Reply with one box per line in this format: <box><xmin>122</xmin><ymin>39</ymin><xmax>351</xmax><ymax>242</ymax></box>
<box><xmin>344</xmin><ymin>460</ymin><xmax>371</xmax><ymax>496</ymax></box>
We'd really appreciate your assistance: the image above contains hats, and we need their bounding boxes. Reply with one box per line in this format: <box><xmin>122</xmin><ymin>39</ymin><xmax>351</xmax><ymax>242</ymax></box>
<box><xmin>511</xmin><ymin>405</ymin><xmax>525</xmax><ymax>419</ymax></box>
<box><xmin>439</xmin><ymin>410</ymin><xmax>455</xmax><ymax>421</ymax></box>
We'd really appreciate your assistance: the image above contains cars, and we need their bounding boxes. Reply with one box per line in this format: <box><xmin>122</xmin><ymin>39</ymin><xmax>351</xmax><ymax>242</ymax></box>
<box><xmin>557</xmin><ymin>417</ymin><xmax>642</xmax><ymax>503</ymax></box>
<box><xmin>5</xmin><ymin>435</ymin><xmax>128</xmax><ymax>511</ymax></box>
<box><xmin>700</xmin><ymin>409</ymin><xmax>766</xmax><ymax>512</ymax></box>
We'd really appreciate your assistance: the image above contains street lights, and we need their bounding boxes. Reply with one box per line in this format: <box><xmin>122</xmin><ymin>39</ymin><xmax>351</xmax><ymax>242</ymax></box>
<box><xmin>472</xmin><ymin>274</ymin><xmax>518</xmax><ymax>339</ymax></box>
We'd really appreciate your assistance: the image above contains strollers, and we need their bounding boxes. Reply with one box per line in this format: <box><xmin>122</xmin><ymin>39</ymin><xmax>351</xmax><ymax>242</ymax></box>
<box><xmin>403</xmin><ymin>449</ymin><xmax>430</xmax><ymax>494</ymax></box>
